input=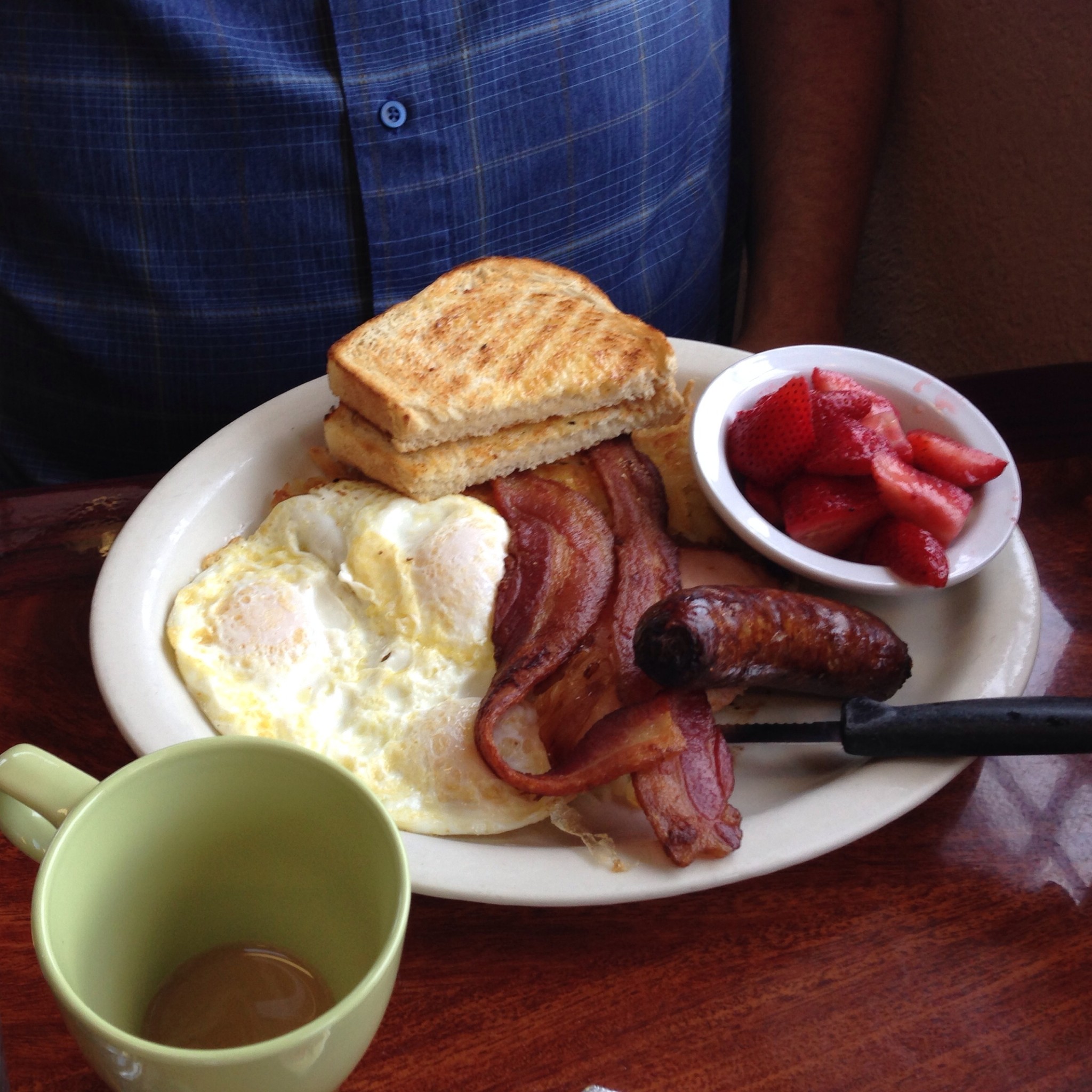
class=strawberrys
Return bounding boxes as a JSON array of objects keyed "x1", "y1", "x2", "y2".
[{"x1": 727, "y1": 365, "x2": 1009, "y2": 587}]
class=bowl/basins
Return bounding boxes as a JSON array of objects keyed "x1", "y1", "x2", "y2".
[{"x1": 688, "y1": 343, "x2": 1022, "y2": 596}]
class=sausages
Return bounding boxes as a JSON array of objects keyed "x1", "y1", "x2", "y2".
[{"x1": 632, "y1": 583, "x2": 913, "y2": 701}]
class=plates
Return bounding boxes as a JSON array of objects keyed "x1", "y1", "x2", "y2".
[{"x1": 88, "y1": 333, "x2": 1042, "y2": 908}]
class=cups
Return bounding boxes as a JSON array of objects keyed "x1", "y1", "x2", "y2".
[{"x1": 0, "y1": 735, "x2": 412, "y2": 1092}]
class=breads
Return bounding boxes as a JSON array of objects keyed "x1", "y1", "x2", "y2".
[{"x1": 322, "y1": 258, "x2": 687, "y2": 503}]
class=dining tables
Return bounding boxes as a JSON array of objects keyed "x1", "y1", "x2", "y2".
[{"x1": 0, "y1": 367, "x2": 1092, "y2": 1092}]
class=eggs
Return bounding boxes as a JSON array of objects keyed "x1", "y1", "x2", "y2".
[{"x1": 166, "y1": 481, "x2": 585, "y2": 836}]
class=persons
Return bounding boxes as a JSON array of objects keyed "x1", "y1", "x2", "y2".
[{"x1": 0, "y1": 0, "x2": 729, "y2": 491}]
